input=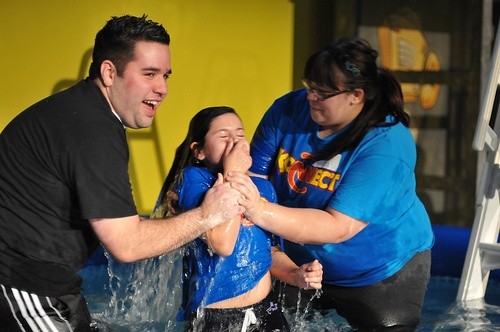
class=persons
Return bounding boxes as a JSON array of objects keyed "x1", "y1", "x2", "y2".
[
  {"x1": 0, "y1": 14, "x2": 247, "y2": 332},
  {"x1": 153, "y1": 106, "x2": 323, "y2": 332},
  {"x1": 224, "y1": 37, "x2": 435, "y2": 332}
]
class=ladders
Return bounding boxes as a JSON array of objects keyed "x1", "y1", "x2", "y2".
[{"x1": 455, "y1": 32, "x2": 500, "y2": 303}]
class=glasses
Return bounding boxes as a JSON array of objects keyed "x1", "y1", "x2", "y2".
[{"x1": 302, "y1": 79, "x2": 355, "y2": 100}]
